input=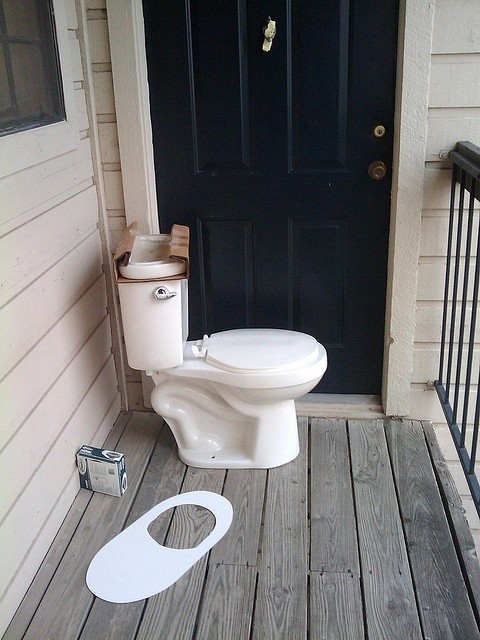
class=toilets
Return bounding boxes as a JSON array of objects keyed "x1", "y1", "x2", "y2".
[{"x1": 112, "y1": 222, "x2": 327, "y2": 469}]
